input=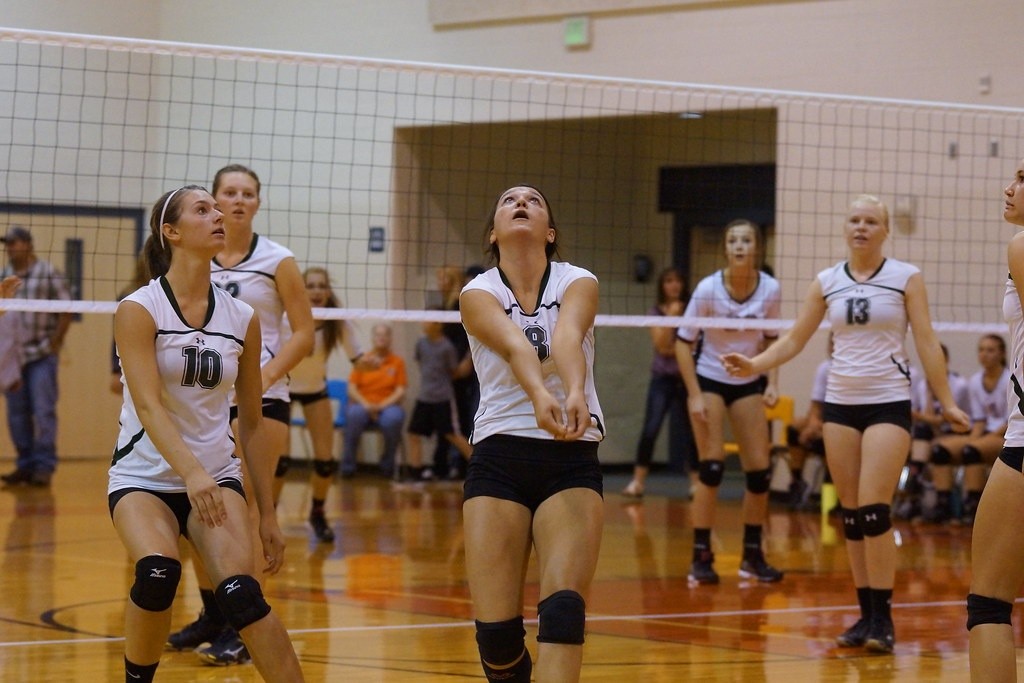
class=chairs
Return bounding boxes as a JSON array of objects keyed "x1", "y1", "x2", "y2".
[
  {"x1": 289, "y1": 383, "x2": 348, "y2": 465},
  {"x1": 724, "y1": 394, "x2": 967, "y2": 525}
]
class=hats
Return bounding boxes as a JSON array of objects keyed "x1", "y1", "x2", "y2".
[{"x1": 0, "y1": 227, "x2": 31, "y2": 242}]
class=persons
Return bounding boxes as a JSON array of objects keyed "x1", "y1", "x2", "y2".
[
  {"x1": 965, "y1": 156, "x2": 1024, "y2": 681},
  {"x1": 898, "y1": 333, "x2": 1011, "y2": 525},
  {"x1": 675, "y1": 219, "x2": 784, "y2": 584},
  {"x1": 107, "y1": 185, "x2": 307, "y2": 683},
  {"x1": 621, "y1": 264, "x2": 703, "y2": 496},
  {"x1": 787, "y1": 325, "x2": 833, "y2": 511},
  {"x1": 407, "y1": 305, "x2": 474, "y2": 478},
  {"x1": 431, "y1": 265, "x2": 487, "y2": 479},
  {"x1": 274, "y1": 259, "x2": 381, "y2": 542},
  {"x1": 718, "y1": 194, "x2": 973, "y2": 655},
  {"x1": 459, "y1": 185, "x2": 606, "y2": 683},
  {"x1": 111, "y1": 246, "x2": 151, "y2": 395},
  {"x1": 166, "y1": 164, "x2": 315, "y2": 659},
  {"x1": 339, "y1": 321, "x2": 407, "y2": 480},
  {"x1": 0, "y1": 226, "x2": 77, "y2": 488}
]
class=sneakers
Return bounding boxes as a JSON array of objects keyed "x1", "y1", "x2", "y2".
[
  {"x1": 688, "y1": 562, "x2": 719, "y2": 585},
  {"x1": 165, "y1": 608, "x2": 224, "y2": 652},
  {"x1": 738, "y1": 558, "x2": 784, "y2": 583},
  {"x1": 836, "y1": 617, "x2": 869, "y2": 648},
  {"x1": 196, "y1": 629, "x2": 253, "y2": 667},
  {"x1": 866, "y1": 616, "x2": 894, "y2": 650}
]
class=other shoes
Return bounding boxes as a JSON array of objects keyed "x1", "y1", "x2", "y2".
[
  {"x1": 911, "y1": 502, "x2": 952, "y2": 527},
  {"x1": 309, "y1": 511, "x2": 334, "y2": 544},
  {"x1": 895, "y1": 499, "x2": 921, "y2": 521},
  {"x1": 33, "y1": 463, "x2": 52, "y2": 486},
  {"x1": 1, "y1": 467, "x2": 32, "y2": 485},
  {"x1": 952, "y1": 505, "x2": 975, "y2": 528}
]
